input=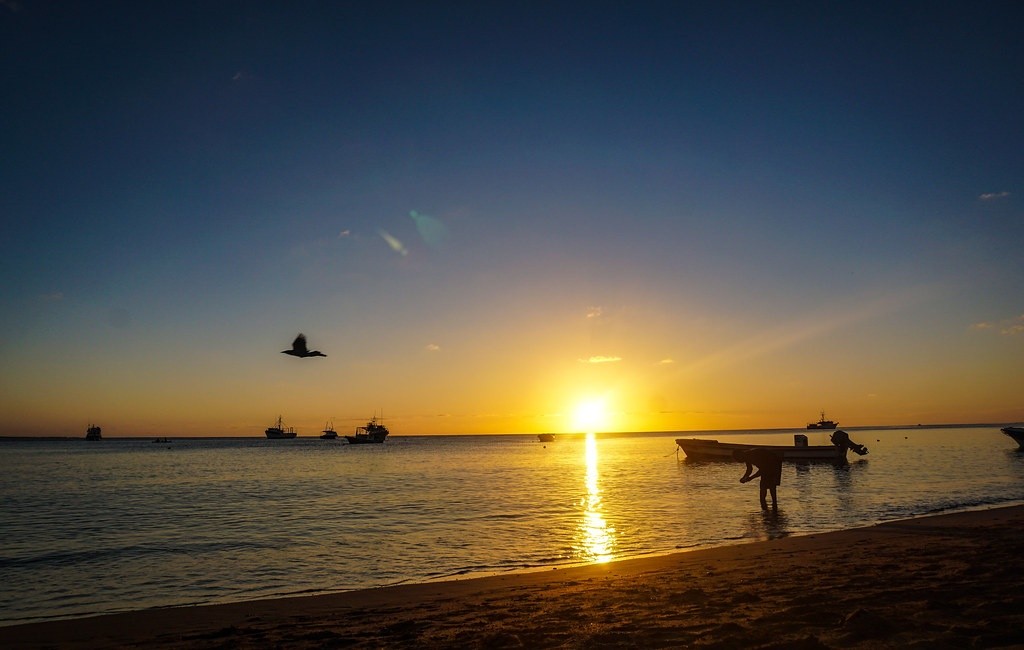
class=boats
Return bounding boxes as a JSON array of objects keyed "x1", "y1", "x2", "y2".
[
  {"x1": 264, "y1": 415, "x2": 297, "y2": 439},
  {"x1": 538, "y1": 433, "x2": 555, "y2": 442},
  {"x1": 152, "y1": 441, "x2": 172, "y2": 443},
  {"x1": 1001, "y1": 427, "x2": 1024, "y2": 451},
  {"x1": 675, "y1": 439, "x2": 848, "y2": 466}
]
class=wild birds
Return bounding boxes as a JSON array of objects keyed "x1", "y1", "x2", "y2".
[{"x1": 280, "y1": 332, "x2": 328, "y2": 358}]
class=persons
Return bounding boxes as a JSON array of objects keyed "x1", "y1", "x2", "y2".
[{"x1": 733, "y1": 447, "x2": 782, "y2": 510}]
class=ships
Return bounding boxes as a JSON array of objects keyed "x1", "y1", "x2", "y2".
[
  {"x1": 86, "y1": 423, "x2": 102, "y2": 441},
  {"x1": 344, "y1": 408, "x2": 389, "y2": 444},
  {"x1": 807, "y1": 411, "x2": 839, "y2": 430},
  {"x1": 319, "y1": 420, "x2": 338, "y2": 439}
]
